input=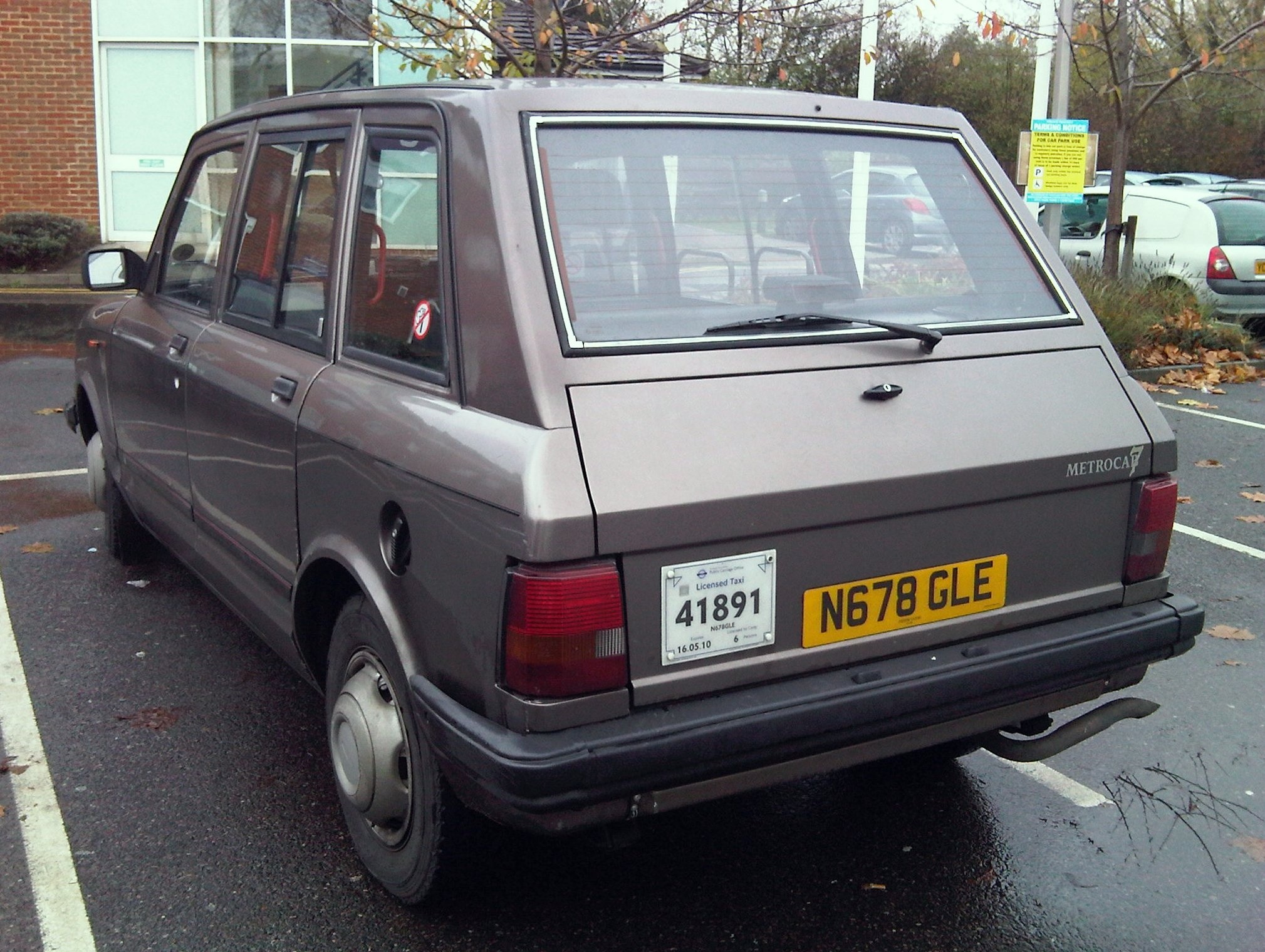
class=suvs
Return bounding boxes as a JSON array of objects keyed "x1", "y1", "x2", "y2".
[
  {"x1": 65, "y1": 78, "x2": 1204, "y2": 911},
  {"x1": 1036, "y1": 186, "x2": 1265, "y2": 345}
]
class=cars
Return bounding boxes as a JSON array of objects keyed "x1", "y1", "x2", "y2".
[
  {"x1": 773, "y1": 163, "x2": 952, "y2": 255},
  {"x1": 1083, "y1": 169, "x2": 1265, "y2": 204}
]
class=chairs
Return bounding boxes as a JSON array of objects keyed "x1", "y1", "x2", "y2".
[{"x1": 548, "y1": 167, "x2": 635, "y2": 298}]
